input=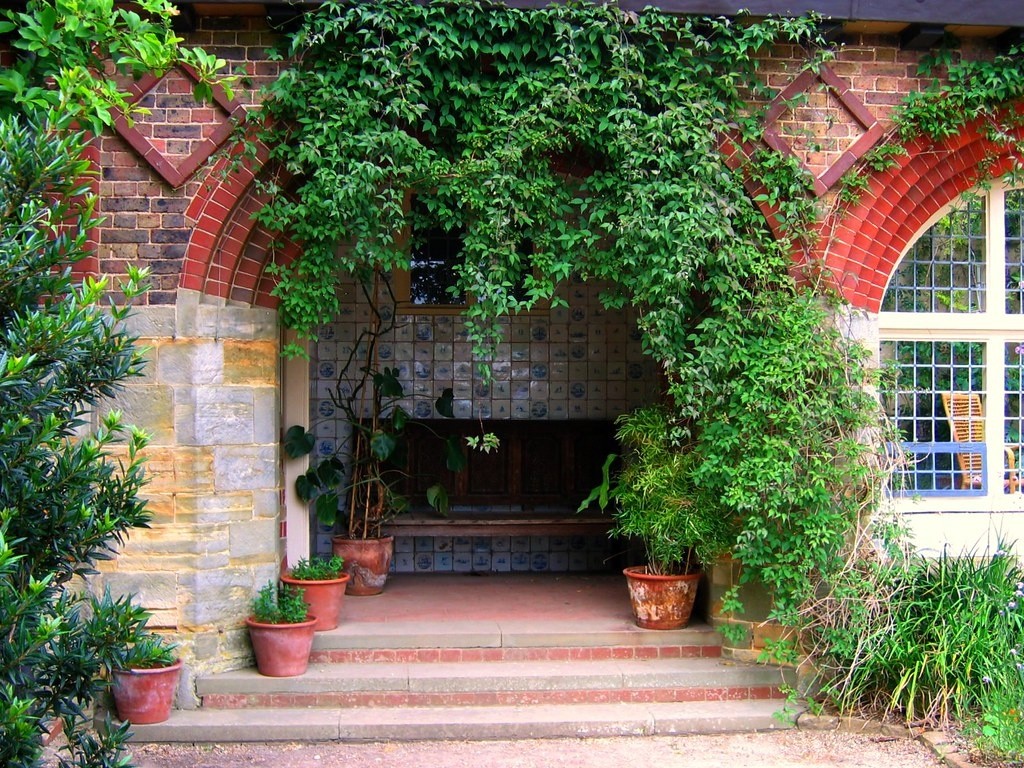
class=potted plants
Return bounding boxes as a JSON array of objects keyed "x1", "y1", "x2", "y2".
[
  {"x1": 284, "y1": 272, "x2": 466, "y2": 596},
  {"x1": 605, "y1": 401, "x2": 741, "y2": 630},
  {"x1": 281, "y1": 553, "x2": 350, "y2": 630},
  {"x1": 244, "y1": 579, "x2": 318, "y2": 677}
]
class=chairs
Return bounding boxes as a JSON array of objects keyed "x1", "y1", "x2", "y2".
[{"x1": 943, "y1": 393, "x2": 1024, "y2": 495}]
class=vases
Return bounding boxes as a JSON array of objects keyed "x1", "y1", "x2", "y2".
[{"x1": 112, "y1": 659, "x2": 182, "y2": 723}]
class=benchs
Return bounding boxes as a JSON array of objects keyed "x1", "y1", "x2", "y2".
[{"x1": 351, "y1": 416, "x2": 616, "y2": 538}]
